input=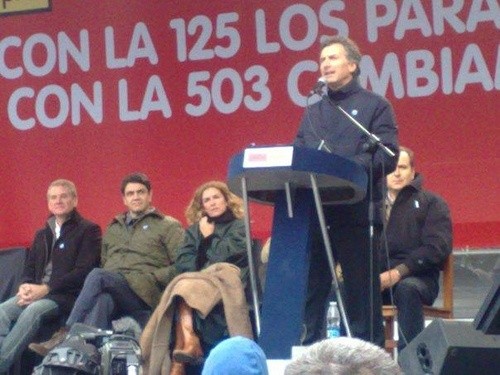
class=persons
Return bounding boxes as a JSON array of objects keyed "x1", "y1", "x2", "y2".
[
  {"x1": 168, "y1": 181, "x2": 254, "y2": 375},
  {"x1": 294, "y1": 36, "x2": 399, "y2": 346},
  {"x1": 201, "y1": 336, "x2": 405, "y2": 375},
  {"x1": 337, "y1": 146, "x2": 454, "y2": 354},
  {"x1": 0, "y1": 179, "x2": 102, "y2": 375},
  {"x1": 28, "y1": 172, "x2": 186, "y2": 356}
]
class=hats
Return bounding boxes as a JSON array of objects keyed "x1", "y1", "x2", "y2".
[{"x1": 201, "y1": 336, "x2": 268, "y2": 375}]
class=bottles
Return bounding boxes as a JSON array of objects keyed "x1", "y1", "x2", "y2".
[{"x1": 327, "y1": 300, "x2": 340, "y2": 338}]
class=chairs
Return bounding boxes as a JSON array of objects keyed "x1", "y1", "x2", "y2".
[
  {"x1": 0, "y1": 237, "x2": 261, "y2": 375},
  {"x1": 380, "y1": 252, "x2": 457, "y2": 354}
]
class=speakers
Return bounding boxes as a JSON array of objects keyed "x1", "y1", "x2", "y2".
[{"x1": 397, "y1": 319, "x2": 500, "y2": 375}]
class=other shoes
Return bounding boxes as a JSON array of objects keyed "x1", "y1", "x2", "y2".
[{"x1": 29, "y1": 327, "x2": 67, "y2": 357}]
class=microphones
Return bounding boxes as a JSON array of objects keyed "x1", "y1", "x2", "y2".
[{"x1": 309, "y1": 77, "x2": 327, "y2": 97}]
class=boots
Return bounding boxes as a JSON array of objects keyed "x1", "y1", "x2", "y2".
[
  {"x1": 168, "y1": 312, "x2": 185, "y2": 375},
  {"x1": 172, "y1": 300, "x2": 204, "y2": 366}
]
